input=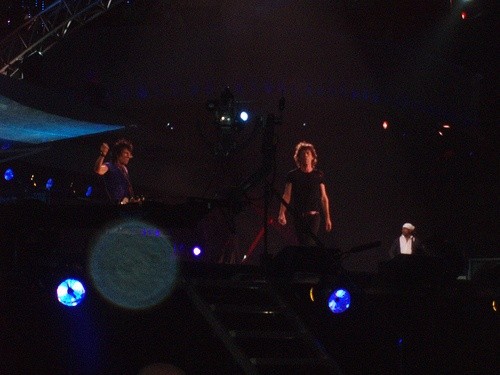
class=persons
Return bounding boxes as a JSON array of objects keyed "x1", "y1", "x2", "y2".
[
  {"x1": 388, "y1": 221, "x2": 426, "y2": 259},
  {"x1": 93, "y1": 139, "x2": 139, "y2": 209},
  {"x1": 278, "y1": 141, "x2": 333, "y2": 247}
]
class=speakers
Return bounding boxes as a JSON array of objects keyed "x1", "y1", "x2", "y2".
[{"x1": 274, "y1": 246, "x2": 335, "y2": 275}]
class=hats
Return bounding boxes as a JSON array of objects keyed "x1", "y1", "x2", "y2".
[{"x1": 402, "y1": 223, "x2": 415, "y2": 231}]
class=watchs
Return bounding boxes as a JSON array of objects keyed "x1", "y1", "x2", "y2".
[{"x1": 99, "y1": 151, "x2": 107, "y2": 156}]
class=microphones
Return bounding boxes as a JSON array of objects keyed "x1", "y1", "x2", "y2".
[{"x1": 278, "y1": 96, "x2": 287, "y2": 121}]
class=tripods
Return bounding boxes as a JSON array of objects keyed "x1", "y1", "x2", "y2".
[{"x1": 219, "y1": 115, "x2": 329, "y2": 269}]
